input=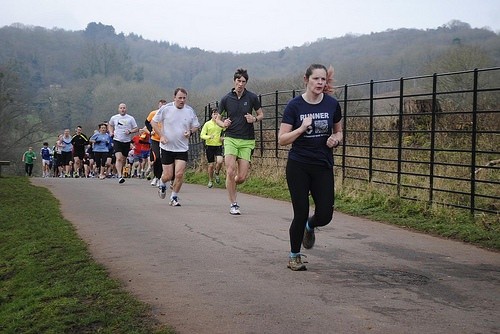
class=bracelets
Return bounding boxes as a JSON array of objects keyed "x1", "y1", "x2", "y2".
[
  {"x1": 334, "y1": 141, "x2": 339, "y2": 148},
  {"x1": 254, "y1": 116, "x2": 257, "y2": 121},
  {"x1": 190, "y1": 130, "x2": 193, "y2": 136},
  {"x1": 129, "y1": 130, "x2": 131, "y2": 134}
]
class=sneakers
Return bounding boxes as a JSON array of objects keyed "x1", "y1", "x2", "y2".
[
  {"x1": 158, "y1": 178, "x2": 166, "y2": 199},
  {"x1": 230, "y1": 202, "x2": 241, "y2": 215},
  {"x1": 287, "y1": 253, "x2": 309, "y2": 271},
  {"x1": 303, "y1": 217, "x2": 315, "y2": 249},
  {"x1": 169, "y1": 195, "x2": 181, "y2": 206}
]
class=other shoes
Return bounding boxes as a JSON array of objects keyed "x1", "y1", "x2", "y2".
[
  {"x1": 53, "y1": 172, "x2": 118, "y2": 180},
  {"x1": 151, "y1": 177, "x2": 158, "y2": 185},
  {"x1": 207, "y1": 180, "x2": 213, "y2": 188},
  {"x1": 169, "y1": 180, "x2": 173, "y2": 189},
  {"x1": 119, "y1": 178, "x2": 125, "y2": 183},
  {"x1": 156, "y1": 179, "x2": 160, "y2": 188},
  {"x1": 214, "y1": 170, "x2": 221, "y2": 185}
]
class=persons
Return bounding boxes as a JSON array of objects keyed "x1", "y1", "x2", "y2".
[
  {"x1": 150, "y1": 87, "x2": 199, "y2": 207},
  {"x1": 40, "y1": 120, "x2": 154, "y2": 180},
  {"x1": 108, "y1": 102, "x2": 138, "y2": 183},
  {"x1": 216, "y1": 68, "x2": 263, "y2": 214},
  {"x1": 278, "y1": 64, "x2": 343, "y2": 270},
  {"x1": 200, "y1": 108, "x2": 226, "y2": 187},
  {"x1": 145, "y1": 100, "x2": 175, "y2": 189},
  {"x1": 22, "y1": 147, "x2": 36, "y2": 176},
  {"x1": 89, "y1": 123, "x2": 110, "y2": 178}
]
